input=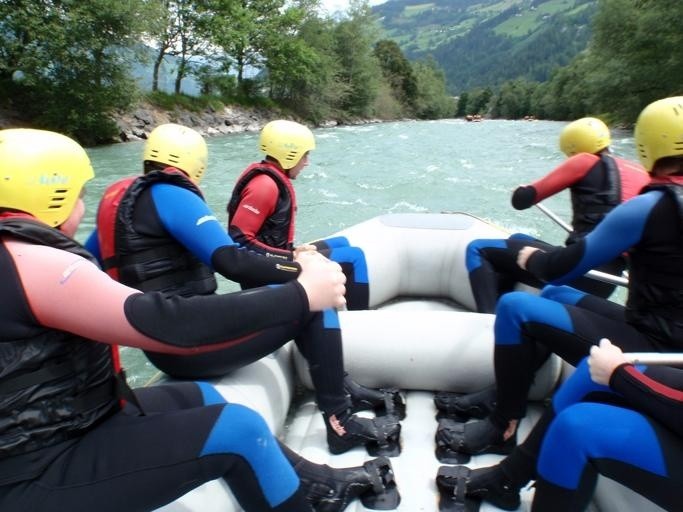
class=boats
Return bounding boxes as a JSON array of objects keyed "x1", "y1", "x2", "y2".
[
  {"x1": 472, "y1": 118, "x2": 480, "y2": 122},
  {"x1": 132, "y1": 206, "x2": 671, "y2": 511},
  {"x1": 528, "y1": 119, "x2": 532, "y2": 121}
]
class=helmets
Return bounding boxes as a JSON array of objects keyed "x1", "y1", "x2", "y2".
[
  {"x1": 260, "y1": 119, "x2": 315, "y2": 170},
  {"x1": 634, "y1": 96, "x2": 683, "y2": 172},
  {"x1": 142, "y1": 123, "x2": 208, "y2": 182},
  {"x1": 0, "y1": 128, "x2": 96, "y2": 227},
  {"x1": 559, "y1": 117, "x2": 610, "y2": 158}
]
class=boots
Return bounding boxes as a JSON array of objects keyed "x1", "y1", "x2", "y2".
[
  {"x1": 322, "y1": 402, "x2": 399, "y2": 455},
  {"x1": 344, "y1": 375, "x2": 401, "y2": 411},
  {"x1": 439, "y1": 409, "x2": 521, "y2": 455},
  {"x1": 439, "y1": 448, "x2": 538, "y2": 511},
  {"x1": 435, "y1": 384, "x2": 496, "y2": 418},
  {"x1": 293, "y1": 458, "x2": 389, "y2": 512}
]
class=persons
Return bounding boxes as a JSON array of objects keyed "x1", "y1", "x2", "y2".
[
  {"x1": 226, "y1": 119, "x2": 371, "y2": 310},
  {"x1": 438, "y1": 342, "x2": 682, "y2": 512},
  {"x1": 1, "y1": 126, "x2": 383, "y2": 512},
  {"x1": 81, "y1": 125, "x2": 401, "y2": 456},
  {"x1": 436, "y1": 97, "x2": 683, "y2": 454},
  {"x1": 467, "y1": 116, "x2": 651, "y2": 313}
]
